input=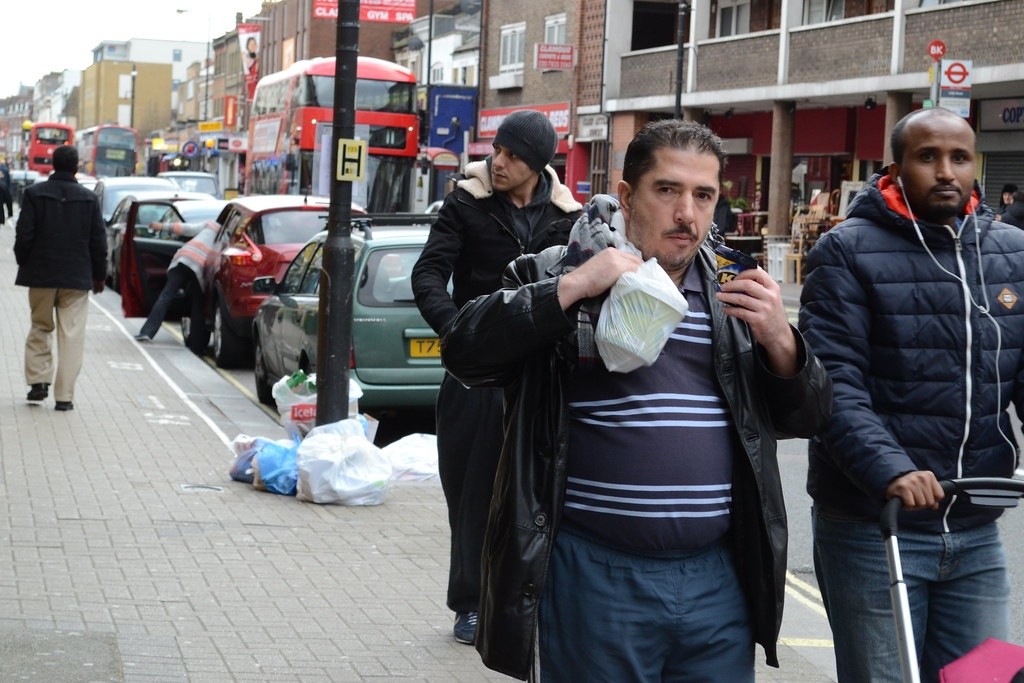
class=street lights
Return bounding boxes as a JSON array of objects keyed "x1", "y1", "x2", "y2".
[{"x1": 176, "y1": 8, "x2": 212, "y2": 173}]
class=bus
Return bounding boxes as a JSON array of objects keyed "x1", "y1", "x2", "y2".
[
  {"x1": 27, "y1": 122, "x2": 73, "y2": 174},
  {"x1": 77, "y1": 124, "x2": 139, "y2": 178},
  {"x1": 244, "y1": 56, "x2": 429, "y2": 225}
]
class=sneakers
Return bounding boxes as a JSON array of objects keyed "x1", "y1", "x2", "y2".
[{"x1": 454, "y1": 612, "x2": 478, "y2": 644}]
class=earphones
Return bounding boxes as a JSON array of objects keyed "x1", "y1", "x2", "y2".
[{"x1": 897, "y1": 176, "x2": 904, "y2": 187}]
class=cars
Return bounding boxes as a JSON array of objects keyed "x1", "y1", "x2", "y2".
[
  {"x1": 6, "y1": 169, "x2": 98, "y2": 201},
  {"x1": 156, "y1": 170, "x2": 225, "y2": 201},
  {"x1": 102, "y1": 192, "x2": 215, "y2": 296},
  {"x1": 91, "y1": 177, "x2": 181, "y2": 224},
  {"x1": 118, "y1": 193, "x2": 366, "y2": 370},
  {"x1": 134, "y1": 197, "x2": 228, "y2": 244},
  {"x1": 254, "y1": 212, "x2": 455, "y2": 420}
]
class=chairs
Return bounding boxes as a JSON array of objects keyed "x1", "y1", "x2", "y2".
[{"x1": 785, "y1": 188, "x2": 846, "y2": 284}]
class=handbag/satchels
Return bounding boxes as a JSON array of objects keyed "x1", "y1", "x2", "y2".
[
  {"x1": 229, "y1": 371, "x2": 392, "y2": 506},
  {"x1": 594, "y1": 243, "x2": 688, "y2": 373}
]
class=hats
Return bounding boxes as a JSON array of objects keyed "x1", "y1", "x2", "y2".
[{"x1": 492, "y1": 110, "x2": 558, "y2": 172}]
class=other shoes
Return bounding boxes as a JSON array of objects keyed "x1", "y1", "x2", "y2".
[
  {"x1": 27, "y1": 383, "x2": 48, "y2": 401},
  {"x1": 135, "y1": 334, "x2": 151, "y2": 342},
  {"x1": 55, "y1": 401, "x2": 74, "y2": 412}
]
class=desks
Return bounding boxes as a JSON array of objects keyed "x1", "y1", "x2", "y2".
[{"x1": 723, "y1": 235, "x2": 762, "y2": 251}]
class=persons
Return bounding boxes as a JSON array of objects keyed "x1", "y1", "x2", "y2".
[
  {"x1": 964, "y1": 179, "x2": 982, "y2": 214},
  {"x1": 435, "y1": 119, "x2": 834, "y2": 683},
  {"x1": 410, "y1": 110, "x2": 583, "y2": 643},
  {"x1": 995, "y1": 184, "x2": 1024, "y2": 230},
  {"x1": 135, "y1": 220, "x2": 232, "y2": 353},
  {"x1": 13, "y1": 144, "x2": 109, "y2": 410},
  {"x1": 0, "y1": 170, "x2": 13, "y2": 224},
  {"x1": 797, "y1": 107, "x2": 1024, "y2": 683},
  {"x1": 245, "y1": 37, "x2": 257, "y2": 67}
]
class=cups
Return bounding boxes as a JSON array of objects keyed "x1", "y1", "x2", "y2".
[{"x1": 597, "y1": 278, "x2": 689, "y2": 367}]
